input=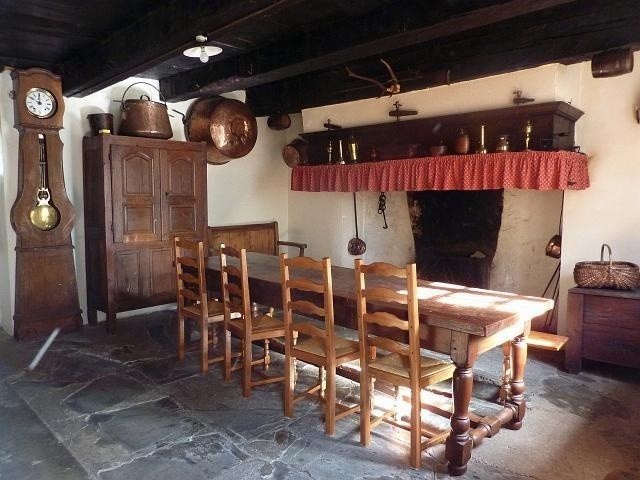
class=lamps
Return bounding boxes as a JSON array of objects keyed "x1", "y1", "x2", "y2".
[{"x1": 182, "y1": 35, "x2": 251, "y2": 63}]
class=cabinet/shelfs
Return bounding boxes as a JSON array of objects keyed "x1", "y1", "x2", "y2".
[
  {"x1": 562, "y1": 280, "x2": 640, "y2": 375},
  {"x1": 82, "y1": 133, "x2": 208, "y2": 336}
]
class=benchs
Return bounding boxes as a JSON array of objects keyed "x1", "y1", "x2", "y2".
[{"x1": 499, "y1": 329, "x2": 568, "y2": 404}]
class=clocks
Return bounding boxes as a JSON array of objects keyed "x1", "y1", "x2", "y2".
[{"x1": 10, "y1": 67, "x2": 66, "y2": 130}]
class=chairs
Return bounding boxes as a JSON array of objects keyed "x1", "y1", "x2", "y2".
[
  {"x1": 279, "y1": 252, "x2": 377, "y2": 437},
  {"x1": 218, "y1": 241, "x2": 299, "y2": 397},
  {"x1": 354, "y1": 258, "x2": 458, "y2": 467},
  {"x1": 207, "y1": 220, "x2": 307, "y2": 258},
  {"x1": 173, "y1": 235, "x2": 251, "y2": 373}
]
